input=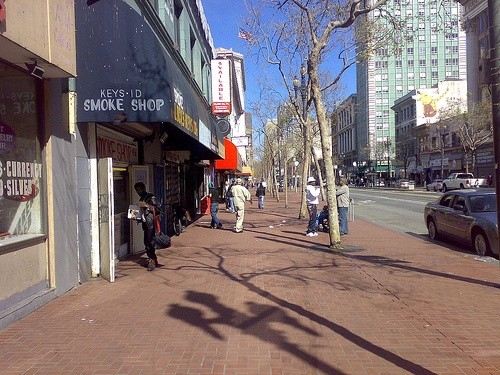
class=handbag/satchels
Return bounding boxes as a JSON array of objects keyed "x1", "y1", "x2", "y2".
[{"x1": 154, "y1": 231, "x2": 171, "y2": 249}]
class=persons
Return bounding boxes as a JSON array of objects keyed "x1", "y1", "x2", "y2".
[
  {"x1": 223, "y1": 181, "x2": 232, "y2": 211},
  {"x1": 336, "y1": 178, "x2": 349, "y2": 235},
  {"x1": 135, "y1": 183, "x2": 161, "y2": 271},
  {"x1": 262, "y1": 180, "x2": 267, "y2": 195},
  {"x1": 232, "y1": 178, "x2": 251, "y2": 232},
  {"x1": 391, "y1": 174, "x2": 401, "y2": 182},
  {"x1": 207, "y1": 183, "x2": 223, "y2": 229},
  {"x1": 383, "y1": 174, "x2": 387, "y2": 184},
  {"x1": 228, "y1": 180, "x2": 236, "y2": 213},
  {"x1": 256, "y1": 183, "x2": 265, "y2": 209},
  {"x1": 425, "y1": 173, "x2": 432, "y2": 191},
  {"x1": 306, "y1": 176, "x2": 322, "y2": 237},
  {"x1": 488, "y1": 173, "x2": 493, "y2": 187}
]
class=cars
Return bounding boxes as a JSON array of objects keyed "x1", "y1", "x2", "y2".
[
  {"x1": 370, "y1": 179, "x2": 415, "y2": 188},
  {"x1": 423, "y1": 190, "x2": 500, "y2": 258},
  {"x1": 426, "y1": 178, "x2": 444, "y2": 192}
]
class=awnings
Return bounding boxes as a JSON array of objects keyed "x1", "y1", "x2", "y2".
[
  {"x1": 214, "y1": 138, "x2": 242, "y2": 173},
  {"x1": 74, "y1": 0, "x2": 224, "y2": 160},
  {"x1": 236, "y1": 165, "x2": 252, "y2": 176}
]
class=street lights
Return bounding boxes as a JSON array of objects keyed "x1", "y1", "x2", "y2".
[
  {"x1": 384, "y1": 135, "x2": 392, "y2": 179},
  {"x1": 293, "y1": 64, "x2": 308, "y2": 138}
]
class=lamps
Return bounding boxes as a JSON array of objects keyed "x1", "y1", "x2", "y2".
[
  {"x1": 158, "y1": 131, "x2": 168, "y2": 143},
  {"x1": 27, "y1": 59, "x2": 45, "y2": 80}
]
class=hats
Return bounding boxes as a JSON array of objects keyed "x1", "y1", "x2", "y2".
[
  {"x1": 208, "y1": 182, "x2": 214, "y2": 188},
  {"x1": 307, "y1": 177, "x2": 316, "y2": 182},
  {"x1": 236, "y1": 178, "x2": 242, "y2": 184}
]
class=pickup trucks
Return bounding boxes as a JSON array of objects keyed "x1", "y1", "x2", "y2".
[{"x1": 442, "y1": 172, "x2": 489, "y2": 192}]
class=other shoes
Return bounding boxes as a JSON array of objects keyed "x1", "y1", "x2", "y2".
[
  {"x1": 340, "y1": 233, "x2": 345, "y2": 236},
  {"x1": 306, "y1": 232, "x2": 315, "y2": 236},
  {"x1": 211, "y1": 223, "x2": 215, "y2": 229},
  {"x1": 147, "y1": 258, "x2": 155, "y2": 270},
  {"x1": 236, "y1": 230, "x2": 243, "y2": 232},
  {"x1": 313, "y1": 232, "x2": 318, "y2": 236},
  {"x1": 216, "y1": 222, "x2": 222, "y2": 229}
]
